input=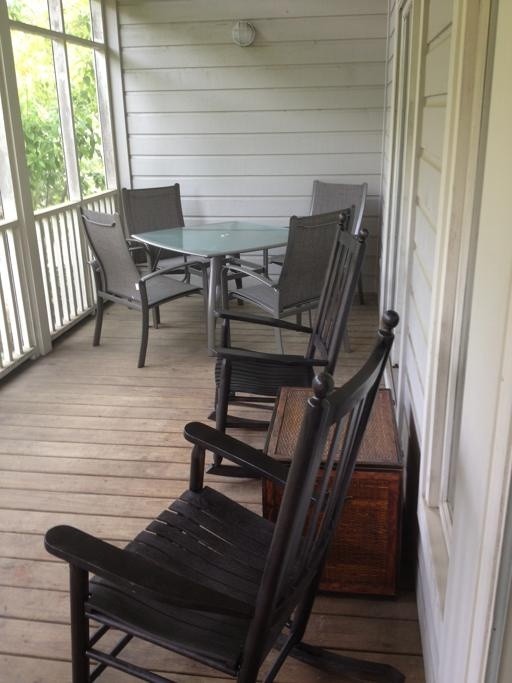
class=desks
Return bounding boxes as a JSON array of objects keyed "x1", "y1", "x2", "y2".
[{"x1": 262, "y1": 385, "x2": 403, "y2": 598}]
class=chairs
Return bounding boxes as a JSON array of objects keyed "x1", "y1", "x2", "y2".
[
  {"x1": 209, "y1": 212, "x2": 368, "y2": 479},
  {"x1": 44, "y1": 311, "x2": 406, "y2": 683}
]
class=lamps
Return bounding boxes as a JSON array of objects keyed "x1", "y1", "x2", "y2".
[{"x1": 232, "y1": 21, "x2": 256, "y2": 47}]
